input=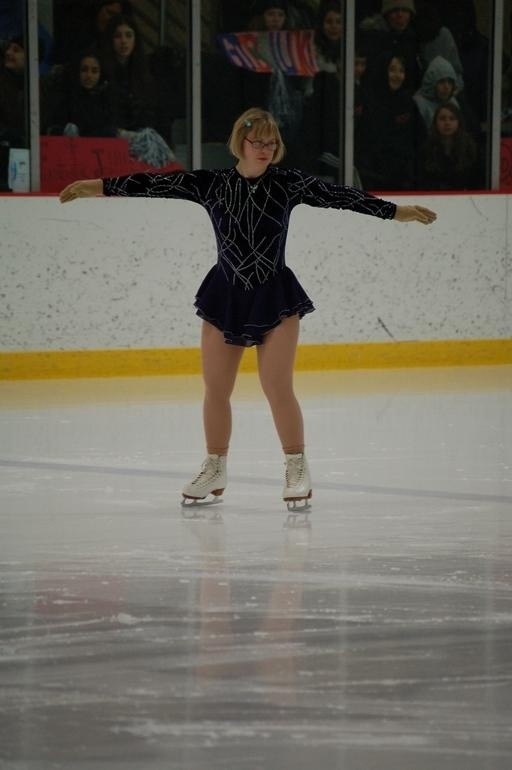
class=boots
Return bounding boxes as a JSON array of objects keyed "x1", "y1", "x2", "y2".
[
  {"x1": 283, "y1": 452, "x2": 312, "y2": 500},
  {"x1": 183, "y1": 454, "x2": 228, "y2": 499}
]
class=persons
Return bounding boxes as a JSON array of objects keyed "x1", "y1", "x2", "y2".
[
  {"x1": 231, "y1": 1, "x2": 490, "y2": 193},
  {"x1": 58, "y1": 108, "x2": 438, "y2": 512},
  {"x1": 1, "y1": 0, "x2": 187, "y2": 168}
]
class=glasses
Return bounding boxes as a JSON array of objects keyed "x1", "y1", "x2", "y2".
[{"x1": 243, "y1": 137, "x2": 279, "y2": 152}]
